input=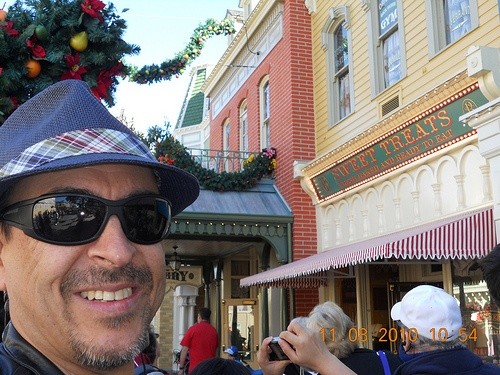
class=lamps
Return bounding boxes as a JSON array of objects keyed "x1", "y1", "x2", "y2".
[{"x1": 167, "y1": 251, "x2": 182, "y2": 272}]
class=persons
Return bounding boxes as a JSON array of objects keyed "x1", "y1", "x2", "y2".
[
  {"x1": 179, "y1": 307, "x2": 219, "y2": 374},
  {"x1": 132, "y1": 321, "x2": 170, "y2": 375},
  {"x1": 256, "y1": 321, "x2": 357, "y2": 374},
  {"x1": 481, "y1": 243, "x2": 500, "y2": 311},
  {"x1": 225, "y1": 346, "x2": 242, "y2": 364},
  {"x1": 0, "y1": 79, "x2": 200, "y2": 375},
  {"x1": 391, "y1": 285, "x2": 500, "y2": 375},
  {"x1": 297, "y1": 301, "x2": 403, "y2": 375}
]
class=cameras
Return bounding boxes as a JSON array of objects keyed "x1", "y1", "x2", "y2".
[{"x1": 269, "y1": 337, "x2": 296, "y2": 360}]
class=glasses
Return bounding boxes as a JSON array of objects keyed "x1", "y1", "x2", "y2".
[
  {"x1": 154, "y1": 333, "x2": 160, "y2": 339},
  {"x1": 0, "y1": 192, "x2": 173, "y2": 246}
]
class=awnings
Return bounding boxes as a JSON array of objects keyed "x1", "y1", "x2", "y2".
[{"x1": 239, "y1": 206, "x2": 496, "y2": 288}]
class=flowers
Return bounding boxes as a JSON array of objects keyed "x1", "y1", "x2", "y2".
[
  {"x1": 6, "y1": 97, "x2": 19, "y2": 111},
  {"x1": 91, "y1": 61, "x2": 125, "y2": 101},
  {"x1": 61, "y1": 55, "x2": 86, "y2": 81},
  {"x1": 26, "y1": 40, "x2": 45, "y2": 58},
  {"x1": 0, "y1": 21, "x2": 19, "y2": 37},
  {"x1": 81, "y1": 0, "x2": 106, "y2": 22},
  {"x1": 241, "y1": 148, "x2": 277, "y2": 171},
  {"x1": 0, "y1": 68, "x2": 3, "y2": 75},
  {"x1": 156, "y1": 155, "x2": 175, "y2": 165}
]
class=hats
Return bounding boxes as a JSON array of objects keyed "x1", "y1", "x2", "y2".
[
  {"x1": 1, "y1": 78, "x2": 200, "y2": 217},
  {"x1": 391, "y1": 284, "x2": 462, "y2": 342},
  {"x1": 224, "y1": 346, "x2": 239, "y2": 355}
]
